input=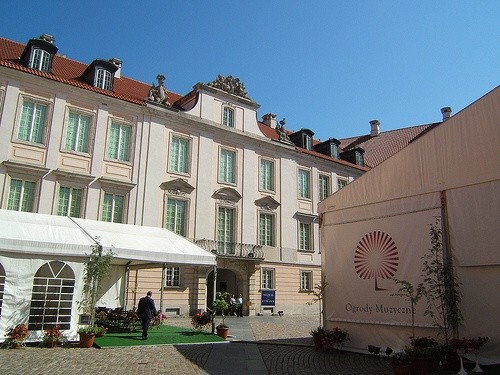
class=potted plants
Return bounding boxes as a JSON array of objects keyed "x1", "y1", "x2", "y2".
[
  {"x1": 440, "y1": 262, "x2": 465, "y2": 368},
  {"x1": 392, "y1": 337, "x2": 439, "y2": 375},
  {"x1": 78, "y1": 327, "x2": 103, "y2": 349},
  {"x1": 213, "y1": 291, "x2": 240, "y2": 339}
]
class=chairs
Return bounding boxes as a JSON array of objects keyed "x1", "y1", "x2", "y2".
[{"x1": 96, "y1": 308, "x2": 162, "y2": 332}]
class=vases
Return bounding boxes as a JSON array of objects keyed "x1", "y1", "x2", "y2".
[
  {"x1": 414, "y1": 344, "x2": 425, "y2": 352},
  {"x1": 45, "y1": 340, "x2": 57, "y2": 349},
  {"x1": 456, "y1": 349, "x2": 469, "y2": 375},
  {"x1": 13, "y1": 343, "x2": 22, "y2": 349},
  {"x1": 313, "y1": 337, "x2": 333, "y2": 350},
  {"x1": 472, "y1": 349, "x2": 485, "y2": 373}
]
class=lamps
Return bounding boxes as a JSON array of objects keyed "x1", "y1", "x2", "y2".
[
  {"x1": 384, "y1": 348, "x2": 393, "y2": 360},
  {"x1": 374, "y1": 348, "x2": 382, "y2": 360},
  {"x1": 369, "y1": 345, "x2": 375, "y2": 358}
]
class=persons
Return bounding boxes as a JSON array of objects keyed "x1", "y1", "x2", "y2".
[
  {"x1": 229, "y1": 293, "x2": 236, "y2": 316},
  {"x1": 137, "y1": 291, "x2": 159, "y2": 340},
  {"x1": 236, "y1": 293, "x2": 243, "y2": 317}
]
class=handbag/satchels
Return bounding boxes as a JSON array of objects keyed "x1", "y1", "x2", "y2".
[{"x1": 138, "y1": 309, "x2": 146, "y2": 318}]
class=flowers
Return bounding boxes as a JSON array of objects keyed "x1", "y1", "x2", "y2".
[
  {"x1": 42, "y1": 325, "x2": 65, "y2": 342},
  {"x1": 4, "y1": 324, "x2": 30, "y2": 342},
  {"x1": 313, "y1": 327, "x2": 346, "y2": 344},
  {"x1": 449, "y1": 336, "x2": 490, "y2": 349},
  {"x1": 409, "y1": 336, "x2": 438, "y2": 347}
]
